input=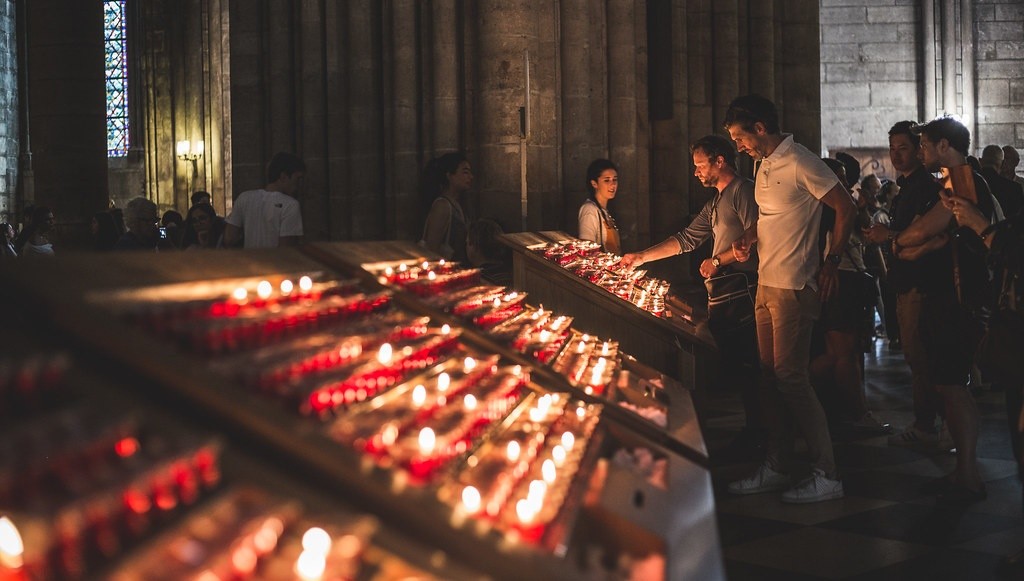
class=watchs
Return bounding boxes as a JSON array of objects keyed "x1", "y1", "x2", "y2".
[
  {"x1": 826, "y1": 254, "x2": 841, "y2": 263},
  {"x1": 712, "y1": 255, "x2": 723, "y2": 270}
]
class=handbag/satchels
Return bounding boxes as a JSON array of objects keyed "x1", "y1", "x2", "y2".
[
  {"x1": 954, "y1": 307, "x2": 985, "y2": 362},
  {"x1": 860, "y1": 272, "x2": 880, "y2": 307},
  {"x1": 973, "y1": 239, "x2": 1023, "y2": 390},
  {"x1": 703, "y1": 272, "x2": 755, "y2": 332},
  {"x1": 417, "y1": 198, "x2": 455, "y2": 259}
]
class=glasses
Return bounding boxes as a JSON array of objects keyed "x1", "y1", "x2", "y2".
[
  {"x1": 191, "y1": 215, "x2": 209, "y2": 224},
  {"x1": 138, "y1": 216, "x2": 161, "y2": 225}
]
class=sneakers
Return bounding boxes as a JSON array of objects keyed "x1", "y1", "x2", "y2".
[
  {"x1": 781, "y1": 469, "x2": 843, "y2": 503},
  {"x1": 887, "y1": 426, "x2": 938, "y2": 446},
  {"x1": 852, "y1": 411, "x2": 894, "y2": 433}
]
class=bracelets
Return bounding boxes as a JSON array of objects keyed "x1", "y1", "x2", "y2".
[
  {"x1": 888, "y1": 229, "x2": 894, "y2": 240},
  {"x1": 980, "y1": 226, "x2": 994, "y2": 241}
]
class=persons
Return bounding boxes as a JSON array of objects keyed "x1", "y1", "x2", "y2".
[
  {"x1": 618, "y1": 135, "x2": 767, "y2": 465},
  {"x1": 0, "y1": 152, "x2": 306, "y2": 259},
  {"x1": 422, "y1": 152, "x2": 513, "y2": 291},
  {"x1": 724, "y1": 95, "x2": 859, "y2": 504},
  {"x1": 809, "y1": 118, "x2": 1024, "y2": 493},
  {"x1": 578, "y1": 158, "x2": 621, "y2": 257}
]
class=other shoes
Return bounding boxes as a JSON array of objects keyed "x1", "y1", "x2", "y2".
[
  {"x1": 727, "y1": 459, "x2": 790, "y2": 496},
  {"x1": 918, "y1": 476, "x2": 952, "y2": 496},
  {"x1": 947, "y1": 479, "x2": 985, "y2": 514}
]
